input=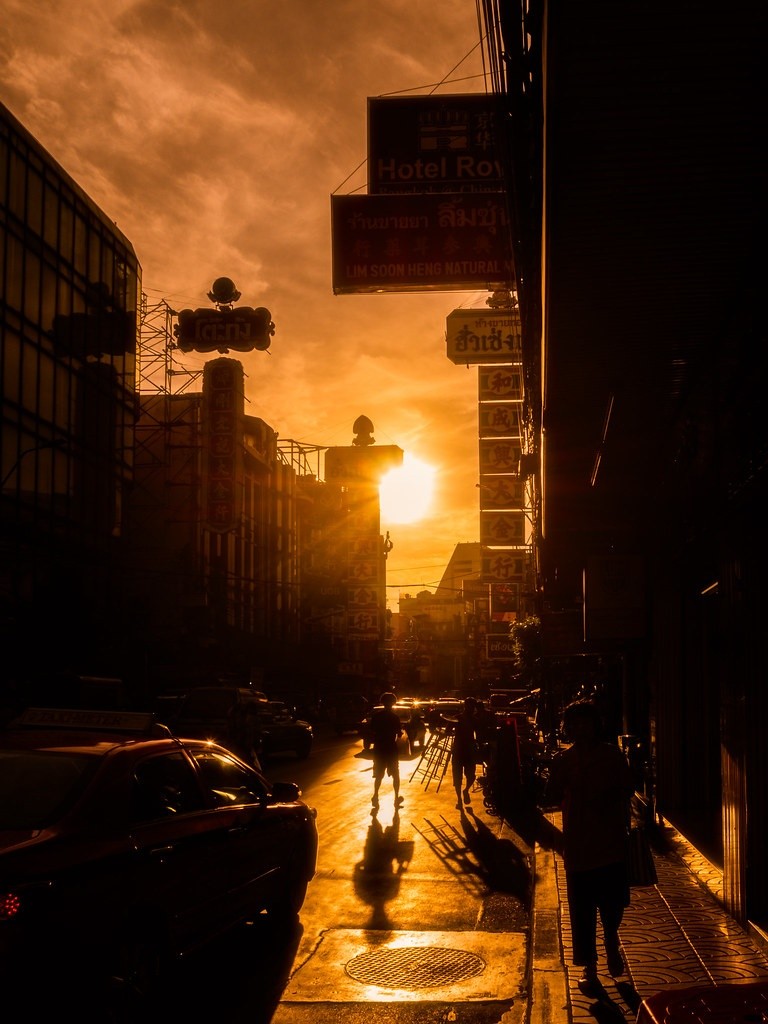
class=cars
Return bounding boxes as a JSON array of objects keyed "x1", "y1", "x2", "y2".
[
  {"x1": 1, "y1": 704, "x2": 316, "y2": 1008},
  {"x1": 354, "y1": 706, "x2": 427, "y2": 753},
  {"x1": 395, "y1": 697, "x2": 493, "y2": 731}
]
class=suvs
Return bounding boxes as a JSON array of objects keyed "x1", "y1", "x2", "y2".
[{"x1": 166, "y1": 686, "x2": 314, "y2": 766}]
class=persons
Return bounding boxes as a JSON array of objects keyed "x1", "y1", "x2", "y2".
[
  {"x1": 542, "y1": 700, "x2": 637, "y2": 995},
  {"x1": 364, "y1": 693, "x2": 403, "y2": 809},
  {"x1": 444, "y1": 698, "x2": 492, "y2": 809}
]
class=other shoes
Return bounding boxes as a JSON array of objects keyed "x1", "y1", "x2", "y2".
[
  {"x1": 578, "y1": 976, "x2": 603, "y2": 997},
  {"x1": 607, "y1": 948, "x2": 624, "y2": 977},
  {"x1": 463, "y1": 790, "x2": 470, "y2": 804}
]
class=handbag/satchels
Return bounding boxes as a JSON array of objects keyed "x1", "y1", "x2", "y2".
[
  {"x1": 395, "y1": 730, "x2": 412, "y2": 757},
  {"x1": 624, "y1": 827, "x2": 659, "y2": 886},
  {"x1": 453, "y1": 735, "x2": 490, "y2": 764}
]
